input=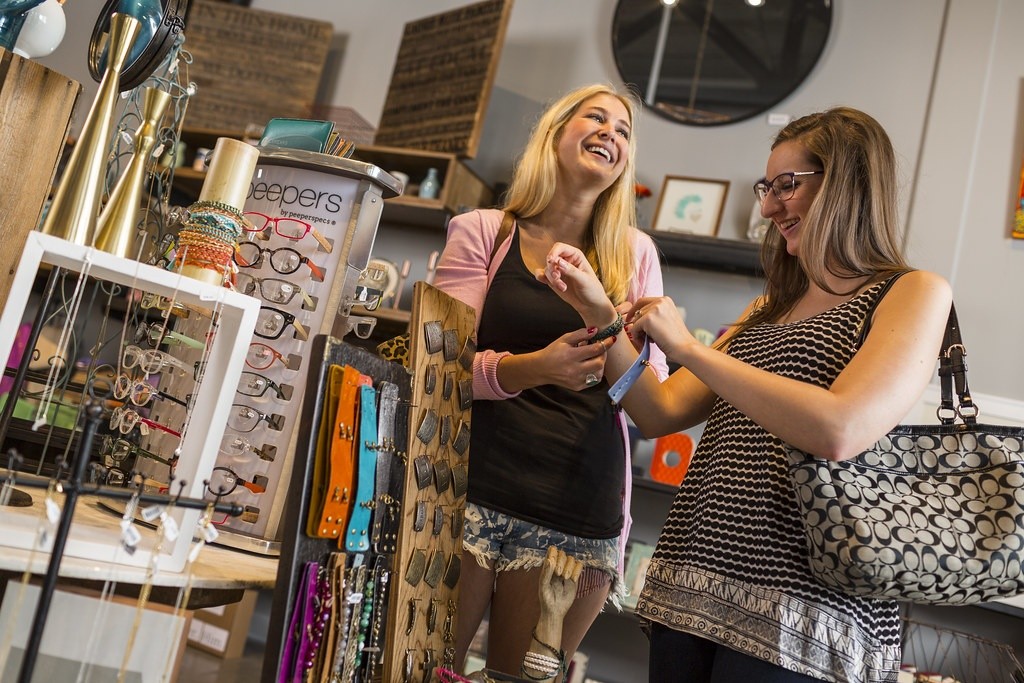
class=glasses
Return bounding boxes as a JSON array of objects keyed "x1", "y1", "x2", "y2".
[
  {"x1": 234, "y1": 272, "x2": 314, "y2": 307},
  {"x1": 186, "y1": 394, "x2": 279, "y2": 432},
  {"x1": 194, "y1": 360, "x2": 286, "y2": 400},
  {"x1": 752, "y1": 171, "x2": 824, "y2": 207},
  {"x1": 242, "y1": 212, "x2": 332, "y2": 254},
  {"x1": 232, "y1": 241, "x2": 324, "y2": 282},
  {"x1": 100, "y1": 434, "x2": 170, "y2": 466},
  {"x1": 122, "y1": 345, "x2": 195, "y2": 374},
  {"x1": 167, "y1": 458, "x2": 266, "y2": 496},
  {"x1": 86, "y1": 461, "x2": 170, "y2": 488},
  {"x1": 205, "y1": 332, "x2": 289, "y2": 370},
  {"x1": 180, "y1": 423, "x2": 274, "y2": 462},
  {"x1": 109, "y1": 406, "x2": 181, "y2": 438},
  {"x1": 113, "y1": 373, "x2": 187, "y2": 406},
  {"x1": 212, "y1": 306, "x2": 307, "y2": 340},
  {"x1": 134, "y1": 205, "x2": 219, "y2": 349},
  {"x1": 159, "y1": 487, "x2": 258, "y2": 525}
]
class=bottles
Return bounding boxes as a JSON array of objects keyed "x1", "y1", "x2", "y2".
[
  {"x1": 12, "y1": 0, "x2": 67, "y2": 60},
  {"x1": 418, "y1": 167, "x2": 440, "y2": 200}
]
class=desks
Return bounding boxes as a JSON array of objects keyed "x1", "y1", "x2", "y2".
[{"x1": 0, "y1": 480, "x2": 279, "y2": 683}]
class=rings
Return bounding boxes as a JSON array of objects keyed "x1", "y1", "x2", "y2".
[
  {"x1": 635, "y1": 309, "x2": 643, "y2": 318},
  {"x1": 601, "y1": 341, "x2": 607, "y2": 351},
  {"x1": 585, "y1": 374, "x2": 598, "y2": 386}
]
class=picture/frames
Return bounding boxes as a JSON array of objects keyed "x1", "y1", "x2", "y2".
[{"x1": 652, "y1": 174, "x2": 732, "y2": 238}]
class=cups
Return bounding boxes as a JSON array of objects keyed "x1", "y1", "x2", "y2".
[{"x1": 390, "y1": 171, "x2": 410, "y2": 197}]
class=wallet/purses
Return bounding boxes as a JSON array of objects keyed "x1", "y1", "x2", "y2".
[{"x1": 258, "y1": 117, "x2": 355, "y2": 159}]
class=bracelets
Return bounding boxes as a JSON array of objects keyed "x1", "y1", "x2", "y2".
[
  {"x1": 607, "y1": 334, "x2": 652, "y2": 404},
  {"x1": 523, "y1": 627, "x2": 568, "y2": 680},
  {"x1": 174, "y1": 200, "x2": 246, "y2": 273},
  {"x1": 594, "y1": 312, "x2": 624, "y2": 342},
  {"x1": 276, "y1": 364, "x2": 399, "y2": 683}
]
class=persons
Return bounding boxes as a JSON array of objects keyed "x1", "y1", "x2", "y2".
[
  {"x1": 625, "y1": 106, "x2": 954, "y2": 683},
  {"x1": 522, "y1": 546, "x2": 583, "y2": 683},
  {"x1": 431, "y1": 82, "x2": 670, "y2": 683}
]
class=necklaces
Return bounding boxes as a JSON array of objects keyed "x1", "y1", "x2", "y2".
[{"x1": 0, "y1": 229, "x2": 201, "y2": 683}]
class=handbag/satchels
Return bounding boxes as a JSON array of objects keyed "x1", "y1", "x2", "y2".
[{"x1": 783, "y1": 270, "x2": 1024, "y2": 606}]
